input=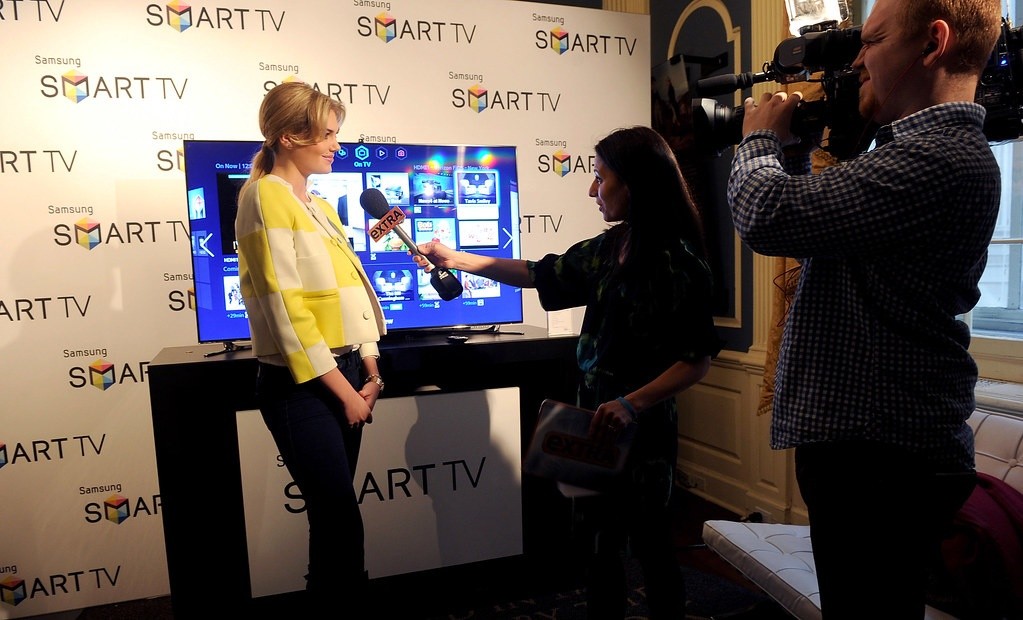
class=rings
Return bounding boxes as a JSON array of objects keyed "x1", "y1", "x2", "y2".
[{"x1": 606, "y1": 425, "x2": 615, "y2": 432}]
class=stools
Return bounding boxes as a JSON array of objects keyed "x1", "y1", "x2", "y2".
[{"x1": 556, "y1": 481, "x2": 601, "y2": 533}]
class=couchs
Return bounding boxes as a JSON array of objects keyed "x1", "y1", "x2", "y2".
[{"x1": 703, "y1": 406, "x2": 1023, "y2": 618}]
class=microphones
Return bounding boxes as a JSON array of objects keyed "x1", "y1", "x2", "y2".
[
  {"x1": 360, "y1": 188, "x2": 464, "y2": 301},
  {"x1": 696, "y1": 72, "x2": 774, "y2": 99}
]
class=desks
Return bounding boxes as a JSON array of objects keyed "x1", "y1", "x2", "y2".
[{"x1": 149, "y1": 335, "x2": 584, "y2": 620}]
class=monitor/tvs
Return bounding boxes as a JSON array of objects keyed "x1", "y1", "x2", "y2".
[{"x1": 183, "y1": 139, "x2": 525, "y2": 357}]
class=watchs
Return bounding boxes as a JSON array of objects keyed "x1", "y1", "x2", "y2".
[{"x1": 370, "y1": 373, "x2": 385, "y2": 391}]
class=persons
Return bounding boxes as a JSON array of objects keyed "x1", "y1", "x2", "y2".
[
  {"x1": 729, "y1": 0, "x2": 1001, "y2": 620},
  {"x1": 236, "y1": 82, "x2": 385, "y2": 620},
  {"x1": 406, "y1": 127, "x2": 719, "y2": 620}
]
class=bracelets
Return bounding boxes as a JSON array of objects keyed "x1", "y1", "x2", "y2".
[
  {"x1": 614, "y1": 395, "x2": 640, "y2": 424},
  {"x1": 366, "y1": 374, "x2": 382, "y2": 383}
]
